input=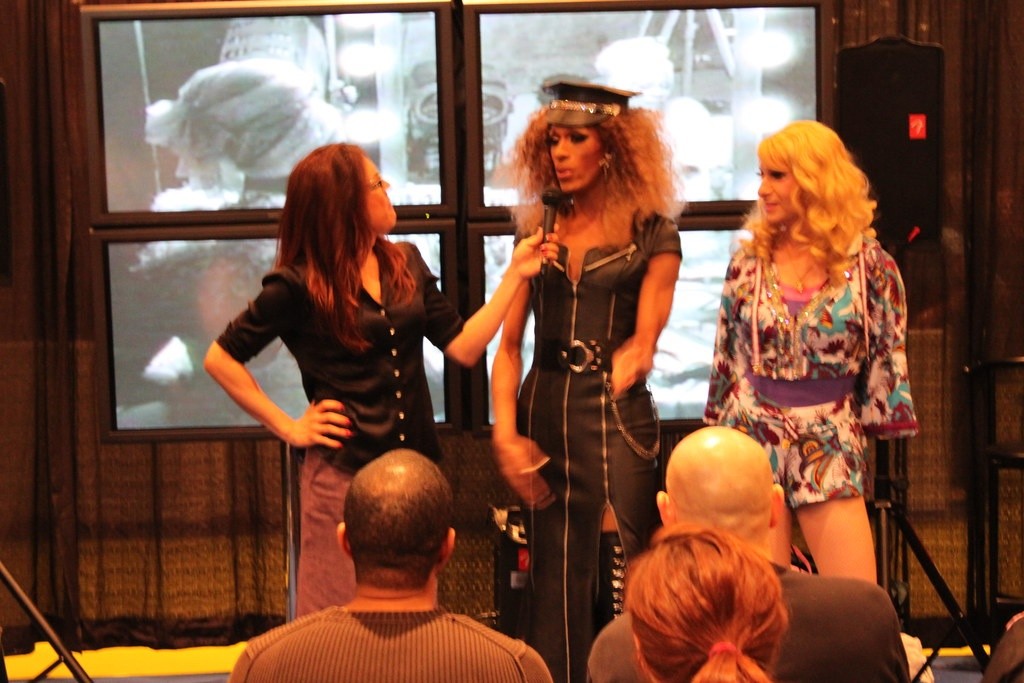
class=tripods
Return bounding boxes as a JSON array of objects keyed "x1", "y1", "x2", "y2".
[{"x1": 861, "y1": 435, "x2": 989, "y2": 668}]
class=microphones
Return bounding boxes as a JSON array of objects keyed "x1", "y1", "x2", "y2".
[{"x1": 540, "y1": 185, "x2": 563, "y2": 276}]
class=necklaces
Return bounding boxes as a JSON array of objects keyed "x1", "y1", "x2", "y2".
[{"x1": 782, "y1": 239, "x2": 815, "y2": 295}]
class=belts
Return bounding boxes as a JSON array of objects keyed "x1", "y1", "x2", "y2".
[{"x1": 532, "y1": 340, "x2": 628, "y2": 374}]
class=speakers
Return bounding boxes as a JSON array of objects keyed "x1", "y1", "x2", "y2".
[{"x1": 833, "y1": 34, "x2": 943, "y2": 244}]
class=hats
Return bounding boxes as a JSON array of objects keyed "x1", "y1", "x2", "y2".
[{"x1": 541, "y1": 73, "x2": 642, "y2": 126}]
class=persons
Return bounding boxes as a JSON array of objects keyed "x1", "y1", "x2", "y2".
[
  {"x1": 227, "y1": 447, "x2": 553, "y2": 682},
  {"x1": 587, "y1": 426, "x2": 911, "y2": 683},
  {"x1": 705, "y1": 120, "x2": 921, "y2": 584},
  {"x1": 490, "y1": 77, "x2": 682, "y2": 683},
  {"x1": 202, "y1": 142, "x2": 559, "y2": 619}
]
